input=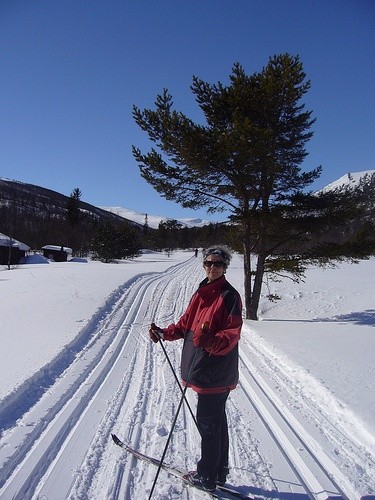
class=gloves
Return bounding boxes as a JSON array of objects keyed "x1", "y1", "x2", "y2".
[
  {"x1": 192, "y1": 327, "x2": 216, "y2": 348},
  {"x1": 148, "y1": 322, "x2": 164, "y2": 344}
]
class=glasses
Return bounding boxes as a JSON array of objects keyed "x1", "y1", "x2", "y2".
[{"x1": 202, "y1": 260, "x2": 226, "y2": 268}]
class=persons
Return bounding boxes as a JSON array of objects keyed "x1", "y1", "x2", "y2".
[
  {"x1": 149, "y1": 245, "x2": 243, "y2": 491},
  {"x1": 195, "y1": 248, "x2": 198, "y2": 257}
]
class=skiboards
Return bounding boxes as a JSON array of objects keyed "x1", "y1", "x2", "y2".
[{"x1": 110, "y1": 432, "x2": 254, "y2": 500}]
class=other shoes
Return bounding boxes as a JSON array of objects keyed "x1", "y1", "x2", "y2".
[
  {"x1": 188, "y1": 467, "x2": 229, "y2": 486},
  {"x1": 182, "y1": 472, "x2": 217, "y2": 492}
]
